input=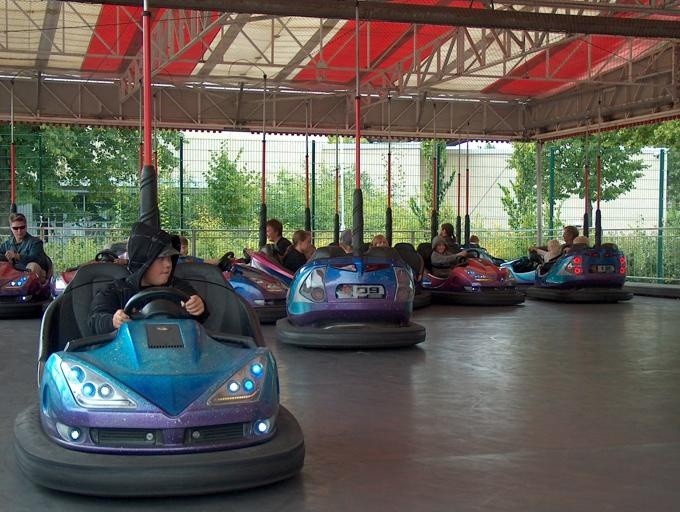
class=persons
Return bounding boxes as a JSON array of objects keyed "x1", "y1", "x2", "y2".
[
  {"x1": 468, "y1": 235, "x2": 479, "y2": 249},
  {"x1": 562, "y1": 226, "x2": 579, "y2": 249},
  {"x1": 88, "y1": 221, "x2": 211, "y2": 335},
  {"x1": 370, "y1": 235, "x2": 390, "y2": 248},
  {"x1": 430, "y1": 235, "x2": 469, "y2": 278},
  {"x1": 265, "y1": 219, "x2": 293, "y2": 266},
  {"x1": 339, "y1": 229, "x2": 353, "y2": 254},
  {"x1": 530, "y1": 240, "x2": 562, "y2": 267},
  {"x1": 438, "y1": 223, "x2": 462, "y2": 254},
  {"x1": 113, "y1": 233, "x2": 130, "y2": 265},
  {"x1": 0, "y1": 212, "x2": 48, "y2": 284},
  {"x1": 281, "y1": 230, "x2": 316, "y2": 273},
  {"x1": 175, "y1": 237, "x2": 219, "y2": 265}
]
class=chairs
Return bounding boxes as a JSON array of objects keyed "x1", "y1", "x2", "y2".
[
  {"x1": 447, "y1": 243, "x2": 463, "y2": 266},
  {"x1": 600, "y1": 243, "x2": 618, "y2": 252},
  {"x1": 364, "y1": 245, "x2": 396, "y2": 263},
  {"x1": 109, "y1": 242, "x2": 126, "y2": 260},
  {"x1": 311, "y1": 245, "x2": 347, "y2": 259},
  {"x1": 57, "y1": 261, "x2": 131, "y2": 351},
  {"x1": 417, "y1": 242, "x2": 433, "y2": 275},
  {"x1": 259, "y1": 244, "x2": 282, "y2": 264},
  {"x1": 170, "y1": 261, "x2": 244, "y2": 351},
  {"x1": 572, "y1": 243, "x2": 590, "y2": 252},
  {"x1": 394, "y1": 243, "x2": 425, "y2": 282}
]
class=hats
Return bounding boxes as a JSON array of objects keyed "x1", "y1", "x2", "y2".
[
  {"x1": 342, "y1": 230, "x2": 352, "y2": 243},
  {"x1": 157, "y1": 241, "x2": 181, "y2": 257},
  {"x1": 573, "y1": 236, "x2": 588, "y2": 244}
]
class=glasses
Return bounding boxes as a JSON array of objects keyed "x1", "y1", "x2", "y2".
[{"x1": 12, "y1": 225, "x2": 25, "y2": 230}]
